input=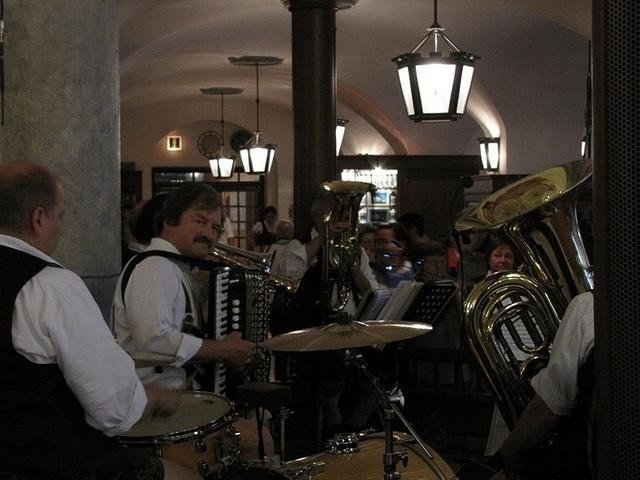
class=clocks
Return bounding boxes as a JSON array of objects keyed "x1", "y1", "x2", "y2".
[{"x1": 198, "y1": 130, "x2": 224, "y2": 158}]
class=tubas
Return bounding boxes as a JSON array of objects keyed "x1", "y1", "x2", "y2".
[
  {"x1": 320, "y1": 181, "x2": 374, "y2": 311},
  {"x1": 454, "y1": 159, "x2": 593, "y2": 432}
]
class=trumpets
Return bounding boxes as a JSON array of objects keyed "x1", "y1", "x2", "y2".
[
  {"x1": 213, "y1": 241, "x2": 276, "y2": 277},
  {"x1": 210, "y1": 243, "x2": 303, "y2": 307}
]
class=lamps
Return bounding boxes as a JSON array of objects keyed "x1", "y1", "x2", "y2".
[
  {"x1": 227, "y1": 55, "x2": 284, "y2": 175},
  {"x1": 336, "y1": 117, "x2": 350, "y2": 157},
  {"x1": 392, "y1": 0, "x2": 482, "y2": 122},
  {"x1": 476, "y1": 137, "x2": 500, "y2": 172},
  {"x1": 580, "y1": 136, "x2": 586, "y2": 157},
  {"x1": 200, "y1": 87, "x2": 243, "y2": 179}
]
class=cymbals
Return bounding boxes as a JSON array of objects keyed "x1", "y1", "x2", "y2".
[{"x1": 257, "y1": 321, "x2": 433, "y2": 351}]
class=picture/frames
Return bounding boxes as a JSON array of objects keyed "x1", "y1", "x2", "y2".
[{"x1": 166, "y1": 135, "x2": 182, "y2": 151}]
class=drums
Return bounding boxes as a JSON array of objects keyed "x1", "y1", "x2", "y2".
[
  {"x1": 208, "y1": 430, "x2": 457, "y2": 479},
  {"x1": 114, "y1": 392, "x2": 240, "y2": 480}
]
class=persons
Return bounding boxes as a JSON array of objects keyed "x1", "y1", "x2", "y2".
[
  {"x1": 106, "y1": 180, "x2": 597, "y2": 480},
  {"x1": 0, "y1": 160, "x2": 147, "y2": 480}
]
